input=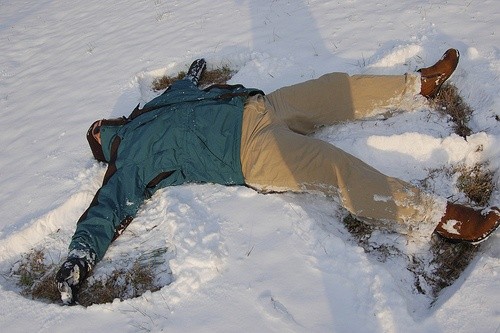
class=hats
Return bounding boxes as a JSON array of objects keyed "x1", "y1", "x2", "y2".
[{"x1": 86, "y1": 118, "x2": 108, "y2": 165}]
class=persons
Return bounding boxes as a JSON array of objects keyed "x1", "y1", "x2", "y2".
[{"x1": 56, "y1": 48, "x2": 500, "y2": 304}]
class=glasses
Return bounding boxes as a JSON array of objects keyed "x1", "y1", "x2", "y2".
[{"x1": 93, "y1": 119, "x2": 102, "y2": 136}]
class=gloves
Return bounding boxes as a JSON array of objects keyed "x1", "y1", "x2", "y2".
[
  {"x1": 51, "y1": 252, "x2": 90, "y2": 306},
  {"x1": 184, "y1": 57, "x2": 207, "y2": 82}
]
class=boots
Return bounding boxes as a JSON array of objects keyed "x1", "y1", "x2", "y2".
[
  {"x1": 434, "y1": 200, "x2": 500, "y2": 246},
  {"x1": 418, "y1": 47, "x2": 461, "y2": 99}
]
